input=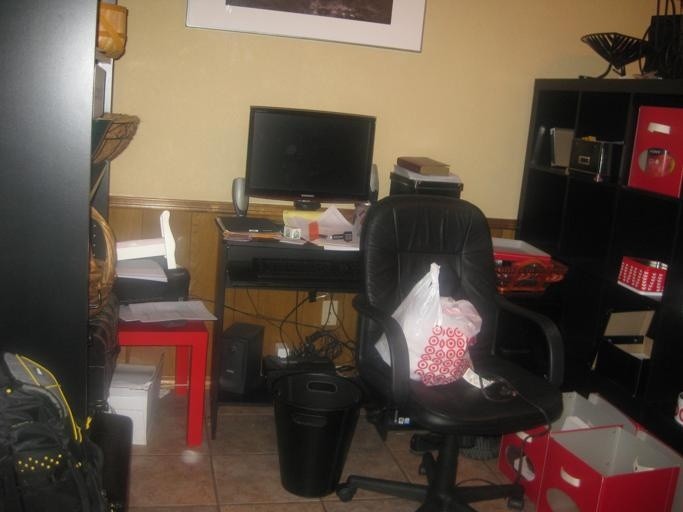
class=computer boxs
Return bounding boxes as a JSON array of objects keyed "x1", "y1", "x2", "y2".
[{"x1": 218, "y1": 321, "x2": 265, "y2": 401}]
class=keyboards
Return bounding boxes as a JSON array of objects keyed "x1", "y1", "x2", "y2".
[{"x1": 251, "y1": 258, "x2": 361, "y2": 284}]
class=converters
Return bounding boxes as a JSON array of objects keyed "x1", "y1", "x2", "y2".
[{"x1": 275, "y1": 341, "x2": 290, "y2": 358}]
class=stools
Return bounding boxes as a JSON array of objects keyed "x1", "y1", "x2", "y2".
[{"x1": 85, "y1": 416, "x2": 132, "y2": 510}]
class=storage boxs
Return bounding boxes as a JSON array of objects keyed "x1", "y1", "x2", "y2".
[
  {"x1": 108, "y1": 351, "x2": 167, "y2": 448},
  {"x1": 580, "y1": 302, "x2": 673, "y2": 413},
  {"x1": 536, "y1": 418, "x2": 679, "y2": 512},
  {"x1": 569, "y1": 137, "x2": 625, "y2": 179},
  {"x1": 498, "y1": 387, "x2": 623, "y2": 509},
  {"x1": 625, "y1": 103, "x2": 682, "y2": 199}
]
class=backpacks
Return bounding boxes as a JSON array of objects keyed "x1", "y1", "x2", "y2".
[{"x1": 0, "y1": 352, "x2": 116, "y2": 512}]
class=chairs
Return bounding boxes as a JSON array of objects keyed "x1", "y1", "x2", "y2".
[{"x1": 335, "y1": 192, "x2": 566, "y2": 511}]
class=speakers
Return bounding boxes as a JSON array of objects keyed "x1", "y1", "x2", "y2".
[
  {"x1": 369, "y1": 164, "x2": 379, "y2": 199},
  {"x1": 231, "y1": 176, "x2": 249, "y2": 217}
]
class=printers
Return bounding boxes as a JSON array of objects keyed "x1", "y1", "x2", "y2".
[{"x1": 389, "y1": 171, "x2": 464, "y2": 204}]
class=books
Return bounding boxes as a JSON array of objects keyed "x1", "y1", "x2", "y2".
[
  {"x1": 214, "y1": 215, "x2": 283, "y2": 242},
  {"x1": 393, "y1": 157, "x2": 462, "y2": 184}
]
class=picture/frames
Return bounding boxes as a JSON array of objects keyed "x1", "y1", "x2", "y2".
[{"x1": 185, "y1": 0, "x2": 427, "y2": 53}]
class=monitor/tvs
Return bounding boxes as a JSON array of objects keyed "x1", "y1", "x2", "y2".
[{"x1": 245, "y1": 105, "x2": 377, "y2": 211}]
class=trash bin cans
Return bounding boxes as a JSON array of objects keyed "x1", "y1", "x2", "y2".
[{"x1": 274, "y1": 371, "x2": 360, "y2": 498}]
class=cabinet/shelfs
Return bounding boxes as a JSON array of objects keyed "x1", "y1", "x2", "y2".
[
  {"x1": 513, "y1": 77, "x2": 683, "y2": 457},
  {"x1": 0, "y1": 1, "x2": 119, "y2": 429}
]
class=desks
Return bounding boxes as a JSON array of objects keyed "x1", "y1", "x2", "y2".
[
  {"x1": 207, "y1": 216, "x2": 551, "y2": 438},
  {"x1": 118, "y1": 319, "x2": 209, "y2": 451}
]
